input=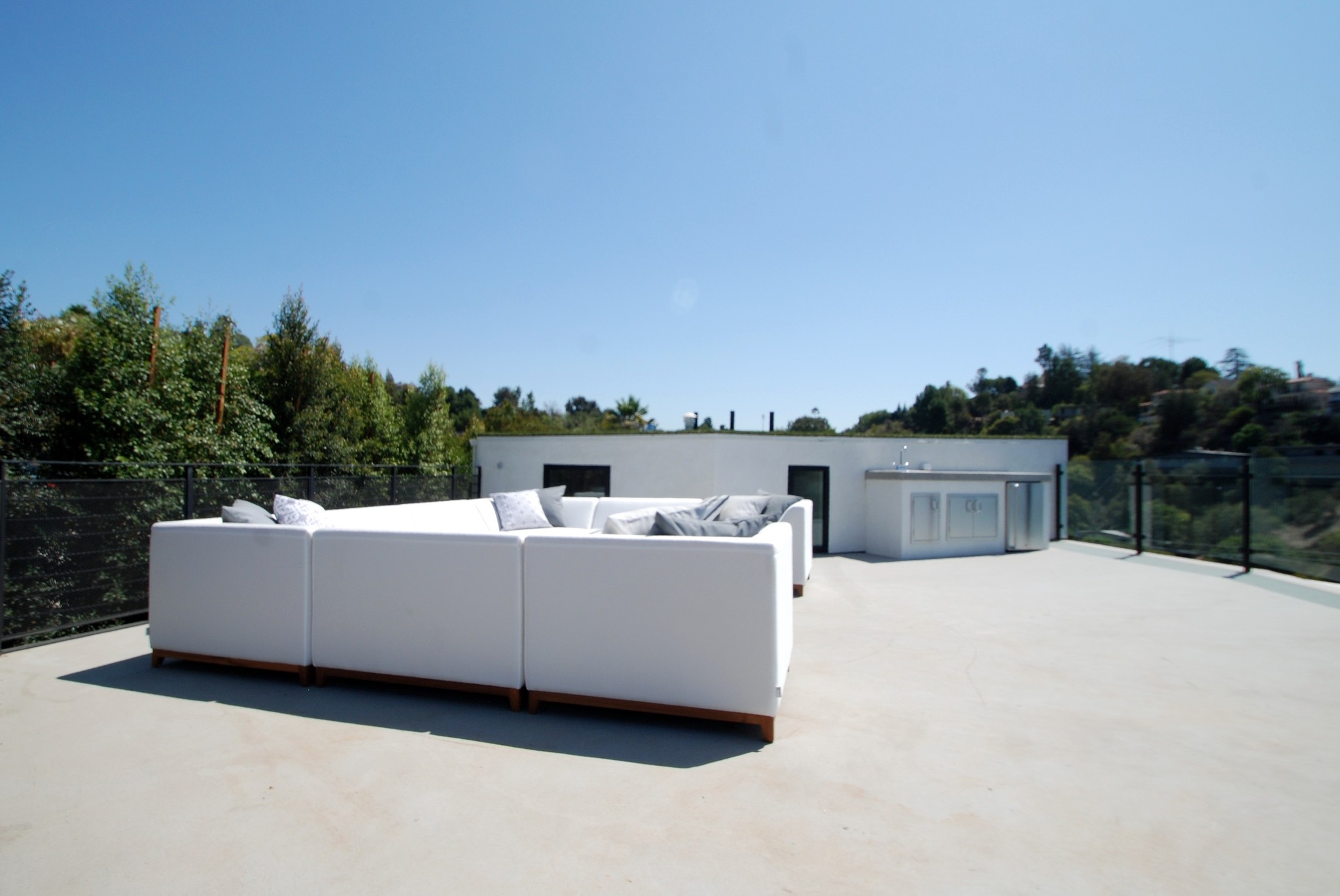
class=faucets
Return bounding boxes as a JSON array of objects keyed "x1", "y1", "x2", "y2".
[{"x1": 896, "y1": 445, "x2": 909, "y2": 470}]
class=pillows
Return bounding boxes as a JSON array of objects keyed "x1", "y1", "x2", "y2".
[{"x1": 535, "y1": 485, "x2": 569, "y2": 526}]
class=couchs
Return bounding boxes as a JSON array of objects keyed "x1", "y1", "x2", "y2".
[{"x1": 144, "y1": 490, "x2": 813, "y2": 747}]
